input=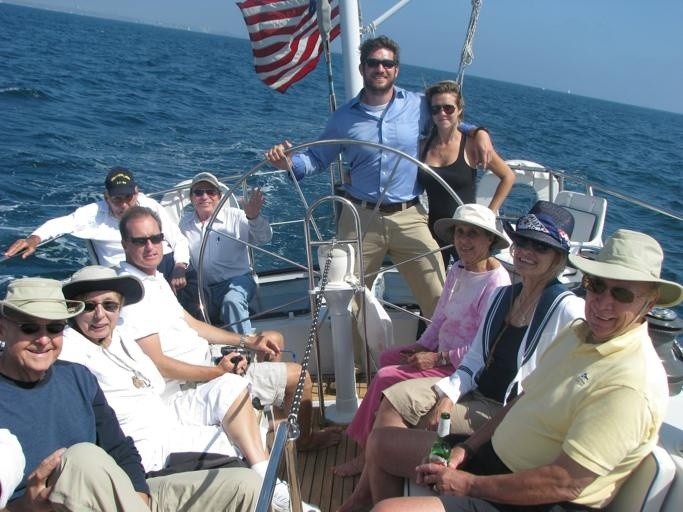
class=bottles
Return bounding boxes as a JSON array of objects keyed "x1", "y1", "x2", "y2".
[{"x1": 429, "y1": 413, "x2": 452, "y2": 475}]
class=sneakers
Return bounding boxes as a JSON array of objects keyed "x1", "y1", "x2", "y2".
[{"x1": 270, "y1": 480, "x2": 318, "y2": 512}]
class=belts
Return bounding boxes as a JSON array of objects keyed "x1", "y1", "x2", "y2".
[{"x1": 348, "y1": 195, "x2": 419, "y2": 212}]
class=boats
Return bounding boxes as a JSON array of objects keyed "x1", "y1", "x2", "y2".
[{"x1": 85, "y1": 0, "x2": 681, "y2": 508}]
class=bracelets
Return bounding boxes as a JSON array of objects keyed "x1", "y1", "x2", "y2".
[
  {"x1": 454, "y1": 443, "x2": 474, "y2": 461},
  {"x1": 239, "y1": 335, "x2": 249, "y2": 348},
  {"x1": 474, "y1": 126, "x2": 490, "y2": 138},
  {"x1": 441, "y1": 352, "x2": 446, "y2": 366}
]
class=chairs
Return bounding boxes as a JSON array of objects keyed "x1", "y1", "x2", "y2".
[
  {"x1": 604, "y1": 443, "x2": 679, "y2": 511},
  {"x1": 551, "y1": 192, "x2": 607, "y2": 289}
]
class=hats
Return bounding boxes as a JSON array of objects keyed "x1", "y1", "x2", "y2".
[
  {"x1": 105, "y1": 167, "x2": 134, "y2": 194},
  {"x1": 190, "y1": 172, "x2": 222, "y2": 198},
  {"x1": 434, "y1": 204, "x2": 510, "y2": 250},
  {"x1": 0, "y1": 277, "x2": 85, "y2": 322},
  {"x1": 568, "y1": 228, "x2": 683, "y2": 308},
  {"x1": 502, "y1": 200, "x2": 575, "y2": 253},
  {"x1": 63, "y1": 265, "x2": 145, "y2": 306}
]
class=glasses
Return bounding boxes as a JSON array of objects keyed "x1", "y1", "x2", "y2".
[
  {"x1": 582, "y1": 275, "x2": 657, "y2": 304},
  {"x1": 363, "y1": 59, "x2": 399, "y2": 68},
  {"x1": 193, "y1": 189, "x2": 215, "y2": 196},
  {"x1": 430, "y1": 105, "x2": 456, "y2": 115},
  {"x1": 3, "y1": 316, "x2": 69, "y2": 335},
  {"x1": 127, "y1": 232, "x2": 164, "y2": 245},
  {"x1": 85, "y1": 301, "x2": 119, "y2": 313},
  {"x1": 514, "y1": 235, "x2": 552, "y2": 255}
]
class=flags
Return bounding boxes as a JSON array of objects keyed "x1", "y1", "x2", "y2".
[{"x1": 235, "y1": 1, "x2": 343, "y2": 95}]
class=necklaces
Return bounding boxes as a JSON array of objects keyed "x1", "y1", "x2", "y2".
[{"x1": 517, "y1": 286, "x2": 543, "y2": 326}]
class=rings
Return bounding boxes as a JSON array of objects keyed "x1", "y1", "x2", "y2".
[
  {"x1": 432, "y1": 485, "x2": 441, "y2": 494},
  {"x1": 424, "y1": 427, "x2": 429, "y2": 430}
]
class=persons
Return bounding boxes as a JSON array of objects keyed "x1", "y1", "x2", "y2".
[
  {"x1": 1, "y1": 278, "x2": 275, "y2": 512},
  {"x1": 264, "y1": 35, "x2": 495, "y2": 394},
  {"x1": 3, "y1": 167, "x2": 213, "y2": 324},
  {"x1": 56, "y1": 266, "x2": 322, "y2": 512},
  {"x1": 116, "y1": 207, "x2": 343, "y2": 454},
  {"x1": 334, "y1": 201, "x2": 583, "y2": 512},
  {"x1": 340, "y1": 79, "x2": 515, "y2": 343},
  {"x1": 176, "y1": 173, "x2": 275, "y2": 334},
  {"x1": 323, "y1": 204, "x2": 512, "y2": 476},
  {"x1": 363, "y1": 227, "x2": 683, "y2": 512}
]
